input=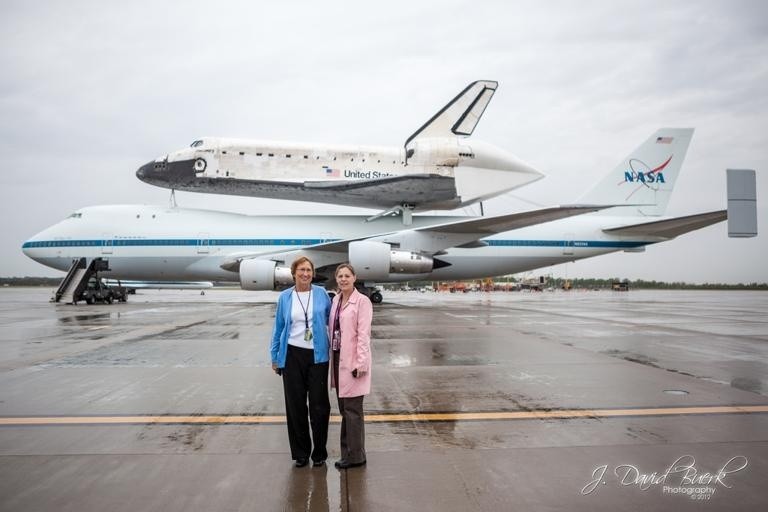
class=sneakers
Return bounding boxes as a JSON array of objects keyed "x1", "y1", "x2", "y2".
[
  {"x1": 296, "y1": 457, "x2": 309, "y2": 467},
  {"x1": 313, "y1": 459, "x2": 325, "y2": 466},
  {"x1": 335, "y1": 459, "x2": 366, "y2": 468}
]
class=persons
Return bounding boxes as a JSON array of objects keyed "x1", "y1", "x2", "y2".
[
  {"x1": 328, "y1": 264, "x2": 373, "y2": 468},
  {"x1": 270, "y1": 256, "x2": 332, "y2": 467}
]
